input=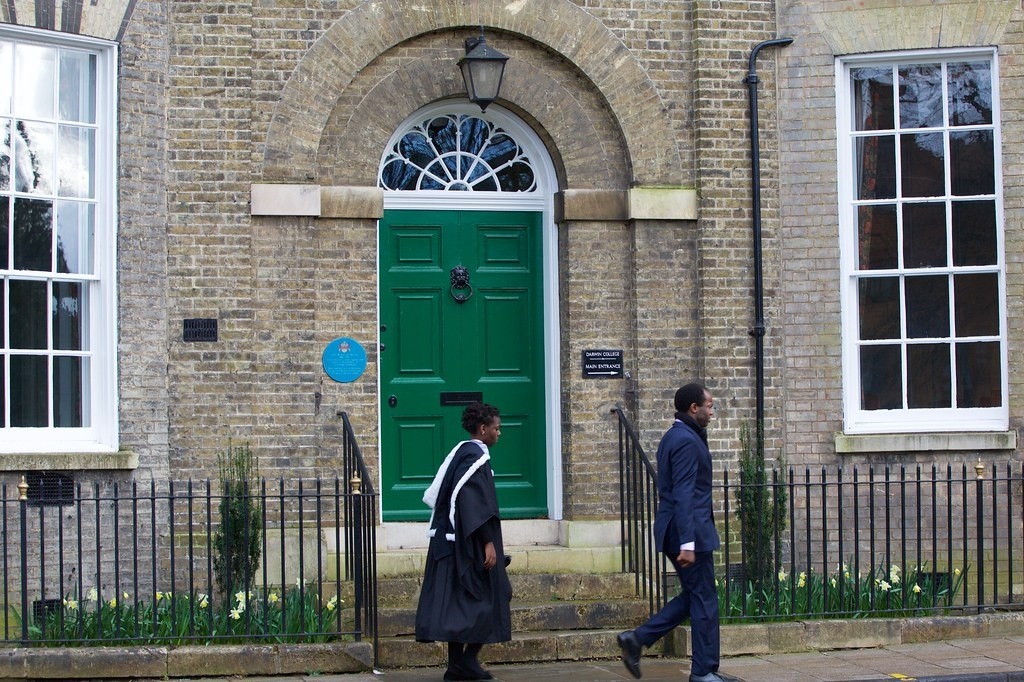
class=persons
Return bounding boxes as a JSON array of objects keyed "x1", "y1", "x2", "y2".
[
  {"x1": 614, "y1": 382, "x2": 725, "y2": 682},
  {"x1": 414, "y1": 405, "x2": 512, "y2": 681}
]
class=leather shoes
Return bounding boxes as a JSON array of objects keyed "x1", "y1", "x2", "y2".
[
  {"x1": 618, "y1": 630, "x2": 641, "y2": 679},
  {"x1": 689, "y1": 672, "x2": 737, "y2": 682}
]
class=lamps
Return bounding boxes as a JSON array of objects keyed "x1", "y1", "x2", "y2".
[{"x1": 454, "y1": 22, "x2": 511, "y2": 115}]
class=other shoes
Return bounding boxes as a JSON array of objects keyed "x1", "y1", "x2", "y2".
[{"x1": 443, "y1": 653, "x2": 493, "y2": 682}]
